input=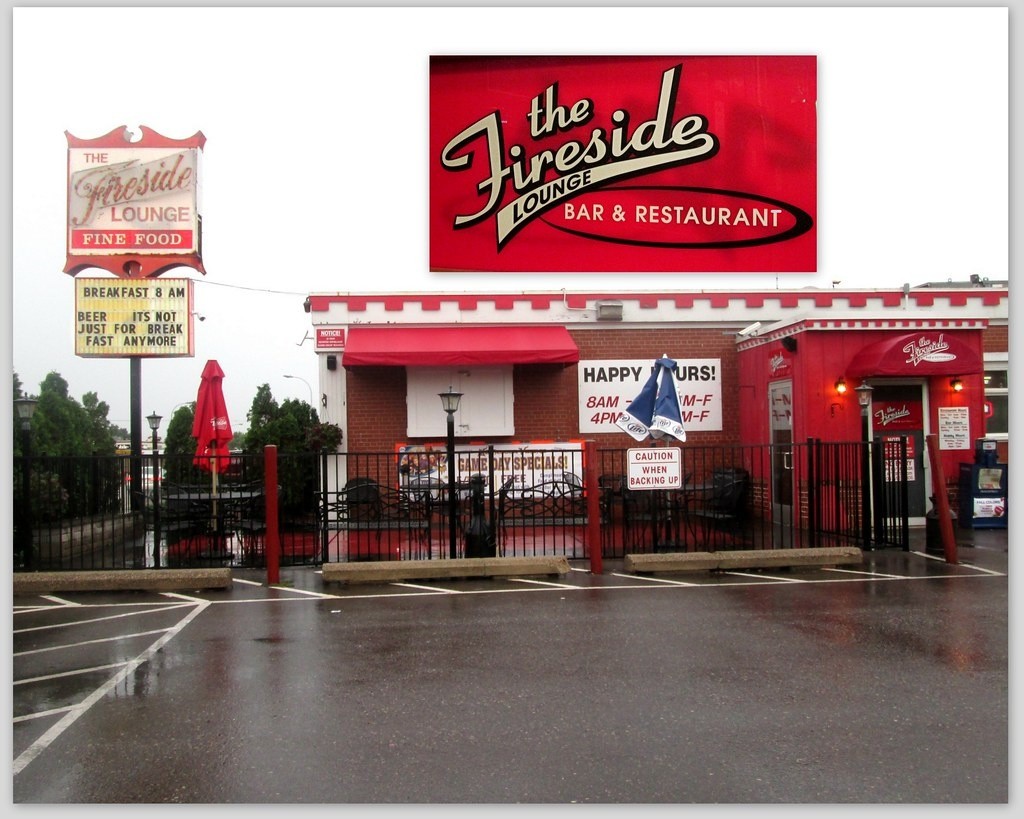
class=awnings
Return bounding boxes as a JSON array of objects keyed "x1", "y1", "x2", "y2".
[
  {"x1": 342, "y1": 325, "x2": 580, "y2": 372},
  {"x1": 848, "y1": 330, "x2": 989, "y2": 378}
]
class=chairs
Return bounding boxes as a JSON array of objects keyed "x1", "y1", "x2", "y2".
[
  {"x1": 464, "y1": 477, "x2": 515, "y2": 545},
  {"x1": 599, "y1": 473, "x2": 632, "y2": 525},
  {"x1": 341, "y1": 476, "x2": 383, "y2": 542},
  {"x1": 130, "y1": 473, "x2": 283, "y2": 562}
]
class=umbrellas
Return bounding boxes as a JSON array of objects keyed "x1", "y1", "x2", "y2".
[
  {"x1": 194, "y1": 360, "x2": 234, "y2": 533},
  {"x1": 615, "y1": 352, "x2": 689, "y2": 532}
]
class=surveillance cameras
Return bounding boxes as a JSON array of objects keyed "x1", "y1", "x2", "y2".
[{"x1": 738, "y1": 321, "x2": 761, "y2": 337}]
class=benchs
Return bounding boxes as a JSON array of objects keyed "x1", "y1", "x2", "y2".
[
  {"x1": 685, "y1": 467, "x2": 750, "y2": 533},
  {"x1": 312, "y1": 483, "x2": 432, "y2": 562}
]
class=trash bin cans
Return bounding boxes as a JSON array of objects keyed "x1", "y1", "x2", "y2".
[{"x1": 710, "y1": 467, "x2": 749, "y2": 515}]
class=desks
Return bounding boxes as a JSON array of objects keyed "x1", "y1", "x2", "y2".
[
  {"x1": 399, "y1": 483, "x2": 472, "y2": 540},
  {"x1": 162, "y1": 492, "x2": 261, "y2": 559},
  {"x1": 657, "y1": 484, "x2": 720, "y2": 550}
]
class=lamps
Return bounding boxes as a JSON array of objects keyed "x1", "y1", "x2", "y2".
[
  {"x1": 782, "y1": 336, "x2": 799, "y2": 351},
  {"x1": 835, "y1": 377, "x2": 847, "y2": 395},
  {"x1": 594, "y1": 299, "x2": 623, "y2": 323},
  {"x1": 950, "y1": 376, "x2": 963, "y2": 392}
]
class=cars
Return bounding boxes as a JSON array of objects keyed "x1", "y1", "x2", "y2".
[{"x1": 125, "y1": 466, "x2": 167, "y2": 491}]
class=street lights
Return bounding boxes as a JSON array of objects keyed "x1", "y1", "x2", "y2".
[
  {"x1": 439, "y1": 387, "x2": 466, "y2": 559},
  {"x1": 282, "y1": 374, "x2": 313, "y2": 412},
  {"x1": 148, "y1": 411, "x2": 163, "y2": 567},
  {"x1": 171, "y1": 402, "x2": 194, "y2": 421}
]
